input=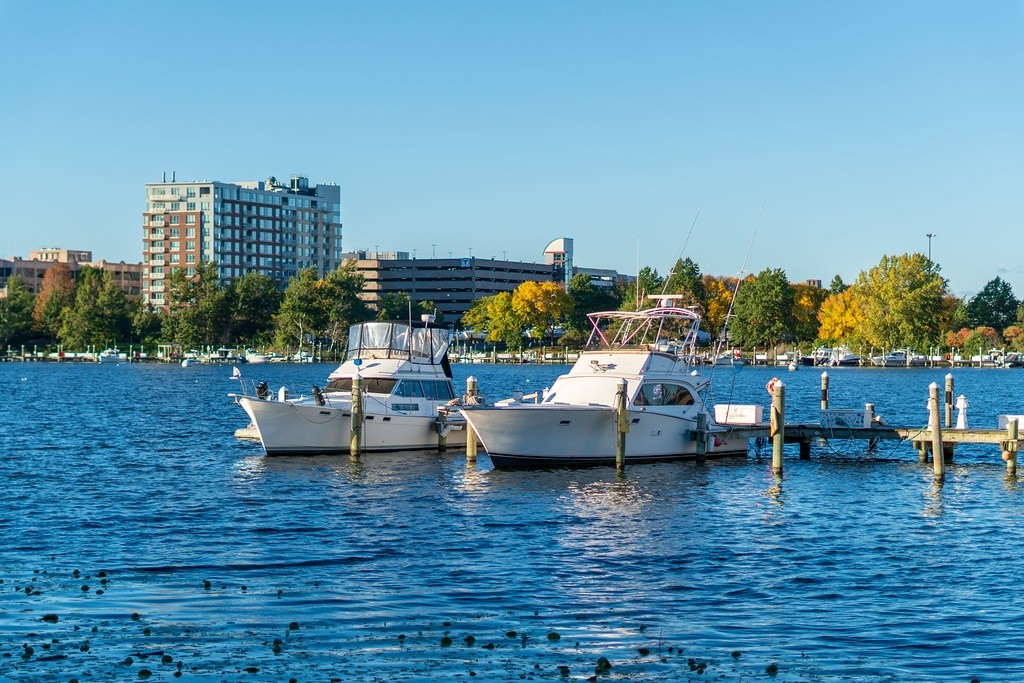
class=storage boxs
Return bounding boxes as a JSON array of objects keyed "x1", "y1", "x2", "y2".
[
  {"x1": 997, "y1": 414, "x2": 1024, "y2": 429},
  {"x1": 821, "y1": 410, "x2": 873, "y2": 428},
  {"x1": 713, "y1": 404, "x2": 765, "y2": 424}
]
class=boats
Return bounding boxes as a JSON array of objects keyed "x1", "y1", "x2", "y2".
[
  {"x1": 228, "y1": 319, "x2": 485, "y2": 456},
  {"x1": 709, "y1": 339, "x2": 1024, "y2": 368},
  {"x1": 435, "y1": 294, "x2": 764, "y2": 473},
  {"x1": 97, "y1": 335, "x2": 272, "y2": 364}
]
